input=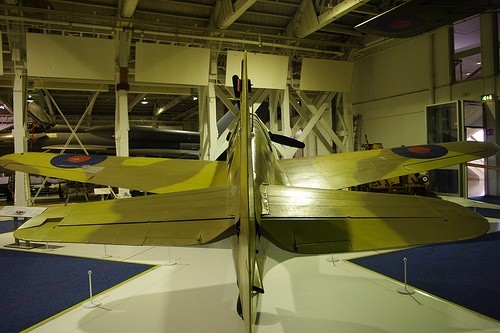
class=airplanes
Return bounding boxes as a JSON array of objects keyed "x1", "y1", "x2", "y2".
[{"x1": 0, "y1": 52, "x2": 500, "y2": 332}]
